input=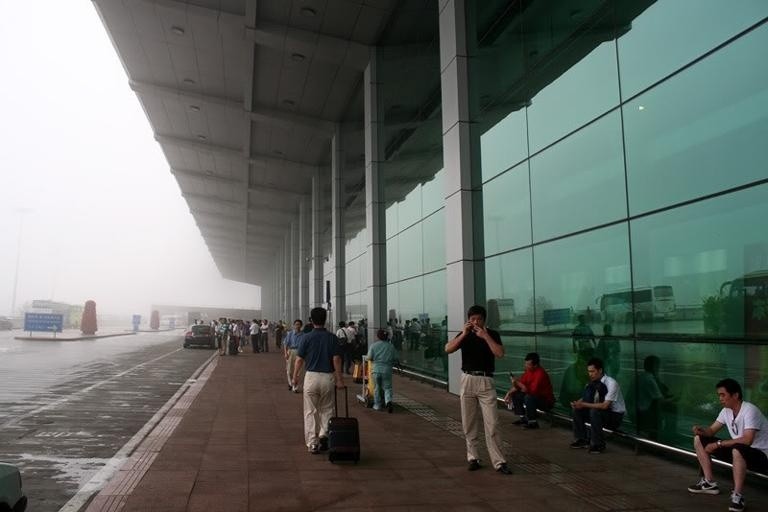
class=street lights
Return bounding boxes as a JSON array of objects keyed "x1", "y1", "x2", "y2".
[{"x1": 10, "y1": 206, "x2": 31, "y2": 317}]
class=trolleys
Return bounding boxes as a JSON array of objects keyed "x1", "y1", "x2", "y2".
[{"x1": 353, "y1": 354, "x2": 375, "y2": 408}]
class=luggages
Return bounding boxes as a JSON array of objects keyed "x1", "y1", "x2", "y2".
[{"x1": 327, "y1": 383, "x2": 361, "y2": 468}]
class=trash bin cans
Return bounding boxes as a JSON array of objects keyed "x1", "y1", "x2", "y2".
[{"x1": 365, "y1": 360, "x2": 384, "y2": 407}]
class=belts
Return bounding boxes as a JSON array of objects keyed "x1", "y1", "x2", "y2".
[{"x1": 462, "y1": 367, "x2": 494, "y2": 379}]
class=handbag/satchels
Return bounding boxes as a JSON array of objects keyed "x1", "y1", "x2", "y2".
[{"x1": 336, "y1": 328, "x2": 348, "y2": 346}]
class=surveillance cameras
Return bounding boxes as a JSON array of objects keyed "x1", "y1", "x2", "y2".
[
  {"x1": 326, "y1": 257, "x2": 329, "y2": 261},
  {"x1": 306, "y1": 257, "x2": 310, "y2": 262}
]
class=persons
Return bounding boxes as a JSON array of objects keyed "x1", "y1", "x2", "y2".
[
  {"x1": 503, "y1": 352, "x2": 556, "y2": 429},
  {"x1": 570, "y1": 357, "x2": 628, "y2": 454},
  {"x1": 358, "y1": 315, "x2": 448, "y2": 357},
  {"x1": 444, "y1": 304, "x2": 512, "y2": 475},
  {"x1": 191, "y1": 317, "x2": 358, "y2": 393},
  {"x1": 291, "y1": 307, "x2": 344, "y2": 453},
  {"x1": 686, "y1": 378, "x2": 768, "y2": 512},
  {"x1": 558, "y1": 314, "x2": 682, "y2": 433},
  {"x1": 366, "y1": 329, "x2": 400, "y2": 414}
]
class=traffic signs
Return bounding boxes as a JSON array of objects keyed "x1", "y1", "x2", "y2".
[
  {"x1": 23, "y1": 313, "x2": 64, "y2": 334},
  {"x1": 543, "y1": 307, "x2": 571, "y2": 327}
]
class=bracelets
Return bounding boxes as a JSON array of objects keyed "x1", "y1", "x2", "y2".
[{"x1": 716, "y1": 440, "x2": 721, "y2": 448}]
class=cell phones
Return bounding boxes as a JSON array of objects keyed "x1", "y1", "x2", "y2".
[{"x1": 510, "y1": 373, "x2": 513, "y2": 377}]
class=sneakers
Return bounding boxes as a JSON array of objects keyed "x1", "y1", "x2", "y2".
[
  {"x1": 287, "y1": 383, "x2": 300, "y2": 393},
  {"x1": 569, "y1": 440, "x2": 607, "y2": 455},
  {"x1": 216, "y1": 347, "x2": 271, "y2": 354},
  {"x1": 727, "y1": 488, "x2": 746, "y2": 512},
  {"x1": 687, "y1": 477, "x2": 721, "y2": 495},
  {"x1": 307, "y1": 435, "x2": 329, "y2": 454},
  {"x1": 372, "y1": 400, "x2": 394, "y2": 413}
]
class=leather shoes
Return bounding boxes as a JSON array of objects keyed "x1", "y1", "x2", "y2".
[
  {"x1": 511, "y1": 416, "x2": 541, "y2": 430},
  {"x1": 496, "y1": 465, "x2": 514, "y2": 475},
  {"x1": 468, "y1": 460, "x2": 482, "y2": 472}
]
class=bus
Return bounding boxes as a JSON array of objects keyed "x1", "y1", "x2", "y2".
[
  {"x1": 484, "y1": 295, "x2": 517, "y2": 323},
  {"x1": 716, "y1": 266, "x2": 768, "y2": 339},
  {"x1": 32, "y1": 299, "x2": 73, "y2": 329},
  {"x1": 594, "y1": 280, "x2": 677, "y2": 324},
  {"x1": 69, "y1": 304, "x2": 86, "y2": 329}
]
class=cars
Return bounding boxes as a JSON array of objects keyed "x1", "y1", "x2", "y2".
[
  {"x1": 0, "y1": 314, "x2": 14, "y2": 333},
  {"x1": 0, "y1": 459, "x2": 29, "y2": 512},
  {"x1": 178, "y1": 322, "x2": 216, "y2": 352}
]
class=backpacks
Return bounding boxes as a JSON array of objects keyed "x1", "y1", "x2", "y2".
[{"x1": 582, "y1": 380, "x2": 608, "y2": 424}]
